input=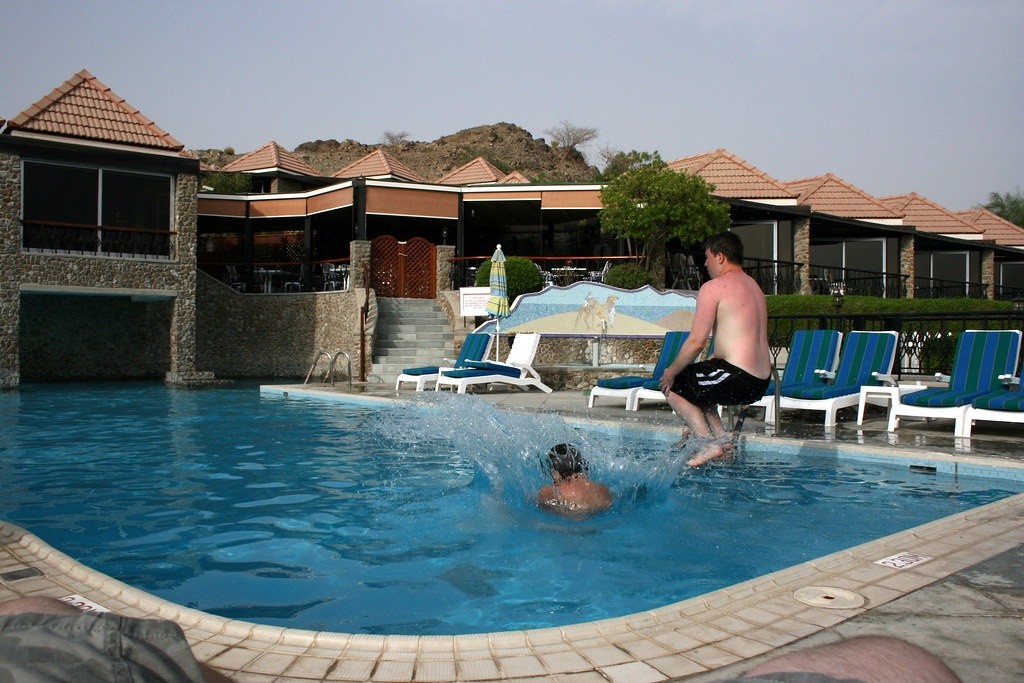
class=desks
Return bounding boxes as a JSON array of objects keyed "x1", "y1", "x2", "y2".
[
  {"x1": 553, "y1": 267, "x2": 587, "y2": 272},
  {"x1": 330, "y1": 268, "x2": 350, "y2": 290},
  {"x1": 254, "y1": 270, "x2": 300, "y2": 294},
  {"x1": 809, "y1": 277, "x2": 825, "y2": 291}
]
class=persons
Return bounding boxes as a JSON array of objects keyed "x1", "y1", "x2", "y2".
[
  {"x1": 537, "y1": 440, "x2": 609, "y2": 520},
  {"x1": 675, "y1": 422, "x2": 733, "y2": 464},
  {"x1": 657, "y1": 231, "x2": 772, "y2": 464}
]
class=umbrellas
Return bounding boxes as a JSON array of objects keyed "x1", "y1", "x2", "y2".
[{"x1": 484, "y1": 243, "x2": 510, "y2": 362}]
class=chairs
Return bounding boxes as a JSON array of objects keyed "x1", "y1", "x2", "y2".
[
  {"x1": 396, "y1": 331, "x2": 495, "y2": 391},
  {"x1": 583, "y1": 260, "x2": 613, "y2": 283},
  {"x1": 585, "y1": 331, "x2": 702, "y2": 412},
  {"x1": 226, "y1": 264, "x2": 249, "y2": 293},
  {"x1": 717, "y1": 329, "x2": 843, "y2": 422},
  {"x1": 963, "y1": 363, "x2": 1024, "y2": 438},
  {"x1": 320, "y1": 263, "x2": 344, "y2": 291},
  {"x1": 824, "y1": 268, "x2": 843, "y2": 295},
  {"x1": 433, "y1": 333, "x2": 553, "y2": 396},
  {"x1": 634, "y1": 337, "x2": 713, "y2": 414},
  {"x1": 285, "y1": 264, "x2": 315, "y2": 292},
  {"x1": 889, "y1": 328, "x2": 1023, "y2": 437},
  {"x1": 771, "y1": 330, "x2": 900, "y2": 427},
  {"x1": 465, "y1": 266, "x2": 478, "y2": 287},
  {"x1": 534, "y1": 263, "x2": 556, "y2": 288}
]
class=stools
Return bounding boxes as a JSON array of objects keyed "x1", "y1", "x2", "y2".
[{"x1": 860, "y1": 385, "x2": 928, "y2": 427}]
